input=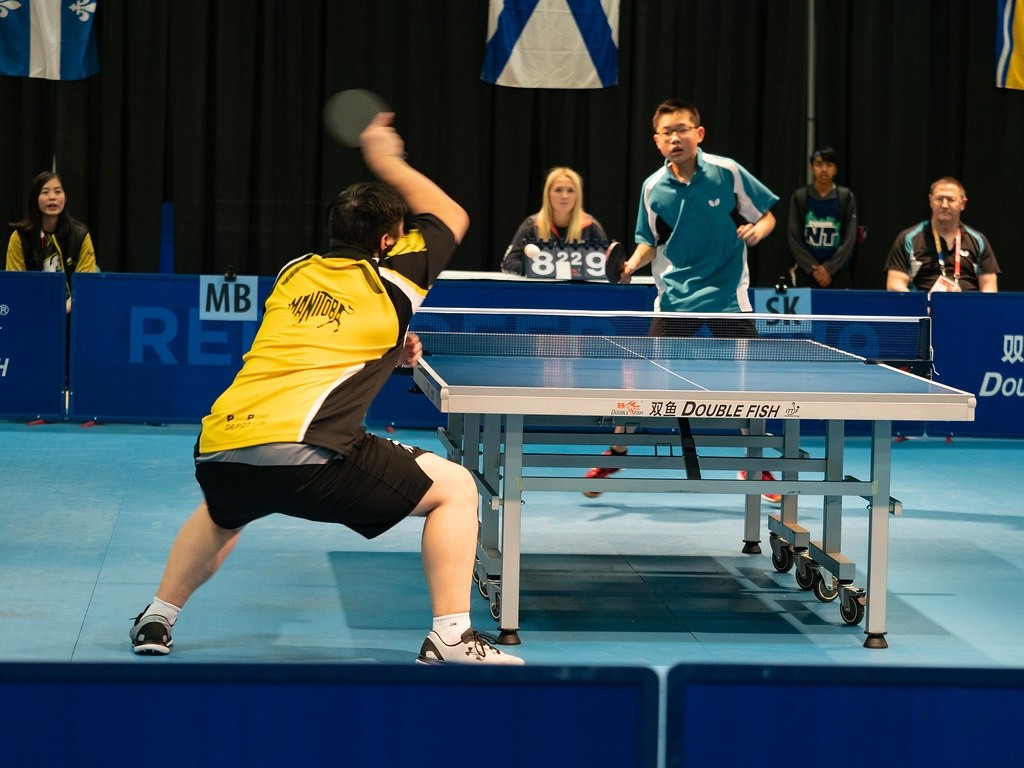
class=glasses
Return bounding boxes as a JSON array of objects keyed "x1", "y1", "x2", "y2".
[{"x1": 656, "y1": 125, "x2": 698, "y2": 138}]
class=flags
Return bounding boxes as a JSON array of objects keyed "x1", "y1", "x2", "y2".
[
  {"x1": 991, "y1": 0, "x2": 1023, "y2": 88},
  {"x1": 0, "y1": 0, "x2": 97, "y2": 80},
  {"x1": 479, "y1": 0, "x2": 620, "y2": 89}
]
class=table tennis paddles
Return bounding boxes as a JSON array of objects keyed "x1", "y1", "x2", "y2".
[
  {"x1": 325, "y1": 90, "x2": 386, "y2": 145},
  {"x1": 603, "y1": 241, "x2": 627, "y2": 283}
]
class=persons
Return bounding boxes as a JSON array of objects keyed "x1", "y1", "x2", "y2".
[
  {"x1": 5, "y1": 170, "x2": 98, "y2": 273},
  {"x1": 128, "y1": 112, "x2": 527, "y2": 666},
  {"x1": 788, "y1": 149, "x2": 859, "y2": 289},
  {"x1": 888, "y1": 177, "x2": 1000, "y2": 293},
  {"x1": 503, "y1": 168, "x2": 610, "y2": 275},
  {"x1": 581, "y1": 101, "x2": 786, "y2": 502}
]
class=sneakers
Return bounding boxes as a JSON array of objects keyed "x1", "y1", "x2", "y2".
[
  {"x1": 129, "y1": 604, "x2": 173, "y2": 655},
  {"x1": 581, "y1": 450, "x2": 625, "y2": 498},
  {"x1": 415, "y1": 627, "x2": 525, "y2": 665},
  {"x1": 737, "y1": 470, "x2": 781, "y2": 503}
]
